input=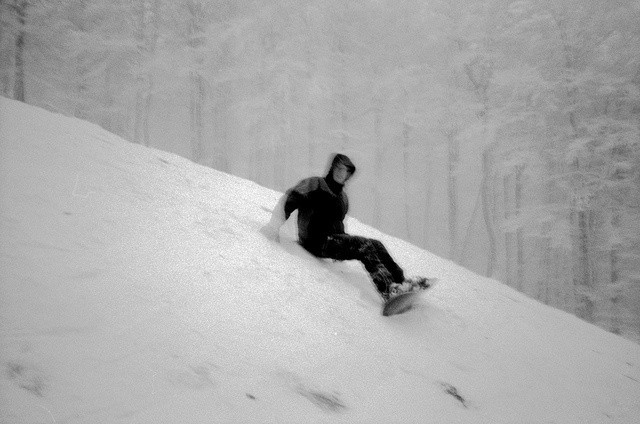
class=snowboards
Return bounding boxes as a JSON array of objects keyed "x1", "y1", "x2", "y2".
[{"x1": 383, "y1": 276, "x2": 430, "y2": 316}]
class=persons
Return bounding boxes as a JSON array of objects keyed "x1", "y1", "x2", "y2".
[{"x1": 260, "y1": 153, "x2": 430, "y2": 316}]
